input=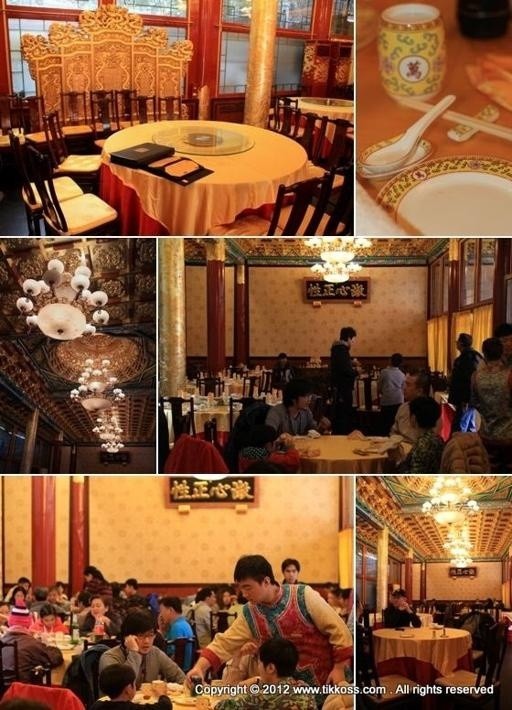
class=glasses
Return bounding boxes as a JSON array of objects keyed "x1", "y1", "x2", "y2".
[{"x1": 138, "y1": 631, "x2": 156, "y2": 640}]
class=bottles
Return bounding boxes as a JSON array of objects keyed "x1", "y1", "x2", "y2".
[
  {"x1": 94, "y1": 614, "x2": 104, "y2": 642},
  {"x1": 70, "y1": 613, "x2": 80, "y2": 645}
]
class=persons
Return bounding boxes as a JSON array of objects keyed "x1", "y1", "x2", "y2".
[
  {"x1": 185, "y1": 555, "x2": 354, "y2": 710},
  {"x1": 324, "y1": 581, "x2": 354, "y2": 635},
  {"x1": 281, "y1": 558, "x2": 306, "y2": 584},
  {"x1": 0, "y1": 564, "x2": 247, "y2": 710},
  {"x1": 224, "y1": 321, "x2": 512, "y2": 474},
  {"x1": 214, "y1": 636, "x2": 320, "y2": 710},
  {"x1": 384, "y1": 589, "x2": 421, "y2": 628}
]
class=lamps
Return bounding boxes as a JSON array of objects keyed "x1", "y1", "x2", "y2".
[
  {"x1": 13, "y1": 257, "x2": 110, "y2": 341},
  {"x1": 102, "y1": 438, "x2": 124, "y2": 454},
  {"x1": 310, "y1": 260, "x2": 364, "y2": 285},
  {"x1": 421, "y1": 477, "x2": 482, "y2": 569},
  {"x1": 70, "y1": 357, "x2": 125, "y2": 413},
  {"x1": 303, "y1": 238, "x2": 374, "y2": 266},
  {"x1": 93, "y1": 414, "x2": 124, "y2": 440}
]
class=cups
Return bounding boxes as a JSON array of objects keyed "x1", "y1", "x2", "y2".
[
  {"x1": 152, "y1": 680, "x2": 164, "y2": 689},
  {"x1": 374, "y1": 3, "x2": 449, "y2": 100}
]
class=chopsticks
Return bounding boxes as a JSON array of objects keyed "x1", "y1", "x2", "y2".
[{"x1": 387, "y1": 88, "x2": 511, "y2": 151}]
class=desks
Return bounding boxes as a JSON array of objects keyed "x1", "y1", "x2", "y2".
[
  {"x1": 91, "y1": 681, "x2": 237, "y2": 710},
  {"x1": 29, "y1": 630, "x2": 93, "y2": 684},
  {"x1": 291, "y1": 363, "x2": 328, "y2": 379},
  {"x1": 284, "y1": 433, "x2": 414, "y2": 473},
  {"x1": 433, "y1": 390, "x2": 449, "y2": 404}
]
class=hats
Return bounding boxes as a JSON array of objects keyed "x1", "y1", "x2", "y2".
[{"x1": 7, "y1": 599, "x2": 33, "y2": 627}]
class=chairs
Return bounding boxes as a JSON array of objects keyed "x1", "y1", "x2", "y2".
[
  {"x1": 430, "y1": 370, "x2": 444, "y2": 380},
  {"x1": 29, "y1": 665, "x2": 52, "y2": 687},
  {"x1": 357, "y1": 599, "x2": 508, "y2": 709},
  {"x1": 0, "y1": 639, "x2": 19, "y2": 690},
  {"x1": 163, "y1": 635, "x2": 197, "y2": 673},
  {"x1": 83, "y1": 638, "x2": 120, "y2": 650},
  {"x1": 355, "y1": 376, "x2": 383, "y2": 416},
  {"x1": 160, "y1": 368, "x2": 282, "y2": 444},
  {"x1": 209, "y1": 610, "x2": 236, "y2": 648}
]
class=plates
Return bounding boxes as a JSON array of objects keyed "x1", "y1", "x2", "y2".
[
  {"x1": 58, "y1": 643, "x2": 74, "y2": 651},
  {"x1": 401, "y1": 633, "x2": 414, "y2": 638},
  {"x1": 372, "y1": 153, "x2": 511, "y2": 236},
  {"x1": 173, "y1": 696, "x2": 197, "y2": 706},
  {"x1": 357, "y1": 139, "x2": 435, "y2": 181}
]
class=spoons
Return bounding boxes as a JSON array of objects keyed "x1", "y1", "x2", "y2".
[{"x1": 358, "y1": 94, "x2": 456, "y2": 173}]
